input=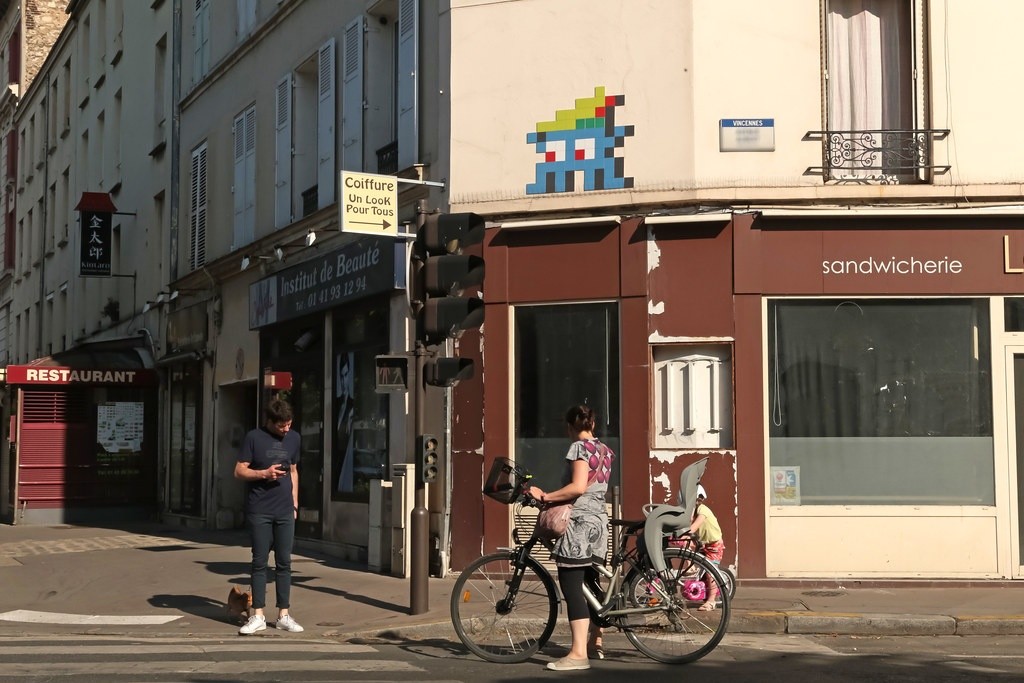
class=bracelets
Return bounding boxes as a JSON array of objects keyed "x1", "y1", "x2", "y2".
[{"x1": 541, "y1": 492, "x2": 547, "y2": 504}]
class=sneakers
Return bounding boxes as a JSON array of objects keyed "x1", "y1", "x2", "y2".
[
  {"x1": 276, "y1": 613, "x2": 304, "y2": 632},
  {"x1": 238, "y1": 613, "x2": 267, "y2": 633}
]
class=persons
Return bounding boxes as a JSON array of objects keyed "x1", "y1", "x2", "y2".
[
  {"x1": 233, "y1": 397, "x2": 305, "y2": 637},
  {"x1": 670, "y1": 482, "x2": 725, "y2": 611},
  {"x1": 522, "y1": 402, "x2": 616, "y2": 671}
]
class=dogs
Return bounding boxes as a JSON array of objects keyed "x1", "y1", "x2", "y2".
[{"x1": 225, "y1": 585, "x2": 252, "y2": 627}]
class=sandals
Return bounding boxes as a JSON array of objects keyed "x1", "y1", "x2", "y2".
[
  {"x1": 682, "y1": 565, "x2": 699, "y2": 577},
  {"x1": 699, "y1": 600, "x2": 718, "y2": 612}
]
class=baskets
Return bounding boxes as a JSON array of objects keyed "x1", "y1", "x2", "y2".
[{"x1": 480, "y1": 455, "x2": 533, "y2": 506}]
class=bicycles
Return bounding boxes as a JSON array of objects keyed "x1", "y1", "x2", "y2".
[
  {"x1": 449, "y1": 456, "x2": 732, "y2": 666},
  {"x1": 629, "y1": 534, "x2": 737, "y2": 615}
]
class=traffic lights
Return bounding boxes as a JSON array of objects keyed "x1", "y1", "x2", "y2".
[{"x1": 411, "y1": 211, "x2": 488, "y2": 389}]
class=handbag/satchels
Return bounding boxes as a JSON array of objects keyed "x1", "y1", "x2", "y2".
[{"x1": 535, "y1": 499, "x2": 574, "y2": 537}]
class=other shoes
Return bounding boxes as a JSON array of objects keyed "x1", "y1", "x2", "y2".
[
  {"x1": 547, "y1": 656, "x2": 591, "y2": 672},
  {"x1": 586, "y1": 648, "x2": 605, "y2": 660}
]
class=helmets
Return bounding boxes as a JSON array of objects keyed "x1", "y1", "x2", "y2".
[{"x1": 696, "y1": 484, "x2": 708, "y2": 500}]
